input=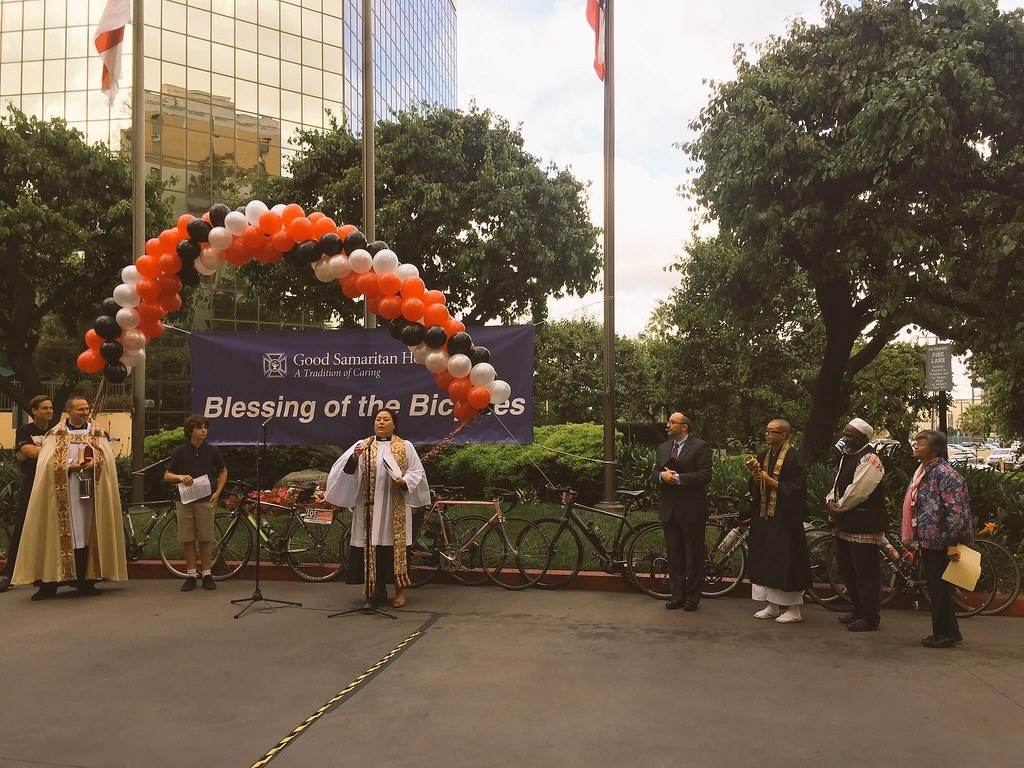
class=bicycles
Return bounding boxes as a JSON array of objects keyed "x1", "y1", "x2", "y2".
[
  {"x1": 339, "y1": 485, "x2": 550, "y2": 591},
  {"x1": 117, "y1": 480, "x2": 223, "y2": 578},
  {"x1": 626, "y1": 492, "x2": 1021, "y2": 618},
  {"x1": 515, "y1": 484, "x2": 662, "y2": 594},
  {"x1": 196, "y1": 478, "x2": 351, "y2": 582}
]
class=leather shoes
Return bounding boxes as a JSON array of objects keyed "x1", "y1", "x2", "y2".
[
  {"x1": 666, "y1": 600, "x2": 683, "y2": 610},
  {"x1": 683, "y1": 601, "x2": 698, "y2": 611}
]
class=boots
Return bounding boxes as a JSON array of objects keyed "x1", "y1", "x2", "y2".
[
  {"x1": 31, "y1": 583, "x2": 57, "y2": 600},
  {"x1": 69, "y1": 548, "x2": 100, "y2": 595}
]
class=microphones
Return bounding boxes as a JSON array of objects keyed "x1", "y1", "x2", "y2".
[
  {"x1": 261, "y1": 417, "x2": 273, "y2": 427},
  {"x1": 365, "y1": 436, "x2": 375, "y2": 448}
]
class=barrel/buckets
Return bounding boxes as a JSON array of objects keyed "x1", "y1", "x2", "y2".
[{"x1": 79, "y1": 468, "x2": 91, "y2": 499}]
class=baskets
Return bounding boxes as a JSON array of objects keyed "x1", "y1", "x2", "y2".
[{"x1": 221, "y1": 481, "x2": 246, "y2": 512}]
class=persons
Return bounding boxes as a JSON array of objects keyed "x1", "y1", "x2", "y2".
[
  {"x1": 326, "y1": 408, "x2": 430, "y2": 608},
  {"x1": 902, "y1": 430, "x2": 973, "y2": 646},
  {"x1": 0, "y1": 395, "x2": 54, "y2": 592},
  {"x1": 653, "y1": 412, "x2": 713, "y2": 611},
  {"x1": 10, "y1": 395, "x2": 128, "y2": 601},
  {"x1": 747, "y1": 419, "x2": 808, "y2": 623},
  {"x1": 163, "y1": 414, "x2": 228, "y2": 592},
  {"x1": 826, "y1": 418, "x2": 885, "y2": 632}
]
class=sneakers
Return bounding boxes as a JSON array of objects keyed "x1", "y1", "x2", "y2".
[
  {"x1": 181, "y1": 577, "x2": 196, "y2": 591},
  {"x1": 203, "y1": 575, "x2": 216, "y2": 590}
]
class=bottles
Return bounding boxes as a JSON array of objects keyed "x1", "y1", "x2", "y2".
[
  {"x1": 259, "y1": 517, "x2": 275, "y2": 537},
  {"x1": 444, "y1": 518, "x2": 457, "y2": 543},
  {"x1": 718, "y1": 526, "x2": 741, "y2": 554},
  {"x1": 881, "y1": 536, "x2": 899, "y2": 561},
  {"x1": 141, "y1": 510, "x2": 160, "y2": 535},
  {"x1": 458, "y1": 527, "x2": 476, "y2": 545},
  {"x1": 587, "y1": 520, "x2": 605, "y2": 543}
]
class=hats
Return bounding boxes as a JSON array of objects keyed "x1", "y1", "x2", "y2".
[{"x1": 848, "y1": 418, "x2": 874, "y2": 441}]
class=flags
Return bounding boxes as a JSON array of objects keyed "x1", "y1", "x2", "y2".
[
  {"x1": 586, "y1": 0, "x2": 605, "y2": 82},
  {"x1": 94, "y1": 0, "x2": 131, "y2": 107}
]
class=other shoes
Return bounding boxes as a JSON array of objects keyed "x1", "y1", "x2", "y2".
[
  {"x1": 0, "y1": 575, "x2": 11, "y2": 592},
  {"x1": 927, "y1": 632, "x2": 963, "y2": 642},
  {"x1": 838, "y1": 612, "x2": 859, "y2": 622},
  {"x1": 923, "y1": 635, "x2": 956, "y2": 648},
  {"x1": 775, "y1": 612, "x2": 802, "y2": 623},
  {"x1": 754, "y1": 605, "x2": 780, "y2": 618},
  {"x1": 393, "y1": 599, "x2": 405, "y2": 608},
  {"x1": 847, "y1": 615, "x2": 881, "y2": 631}
]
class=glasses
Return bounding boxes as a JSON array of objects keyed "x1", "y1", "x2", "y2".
[
  {"x1": 667, "y1": 420, "x2": 687, "y2": 425},
  {"x1": 764, "y1": 429, "x2": 782, "y2": 434}
]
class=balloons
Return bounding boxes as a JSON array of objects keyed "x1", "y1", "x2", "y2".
[{"x1": 76, "y1": 198, "x2": 511, "y2": 421}]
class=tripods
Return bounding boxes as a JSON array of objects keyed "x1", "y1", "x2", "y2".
[
  {"x1": 327, "y1": 447, "x2": 397, "y2": 619},
  {"x1": 230, "y1": 425, "x2": 302, "y2": 619}
]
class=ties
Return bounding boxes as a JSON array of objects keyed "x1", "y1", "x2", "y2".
[{"x1": 671, "y1": 443, "x2": 679, "y2": 460}]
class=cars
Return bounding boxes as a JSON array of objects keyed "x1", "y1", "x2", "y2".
[{"x1": 948, "y1": 441, "x2": 1024, "y2": 472}]
